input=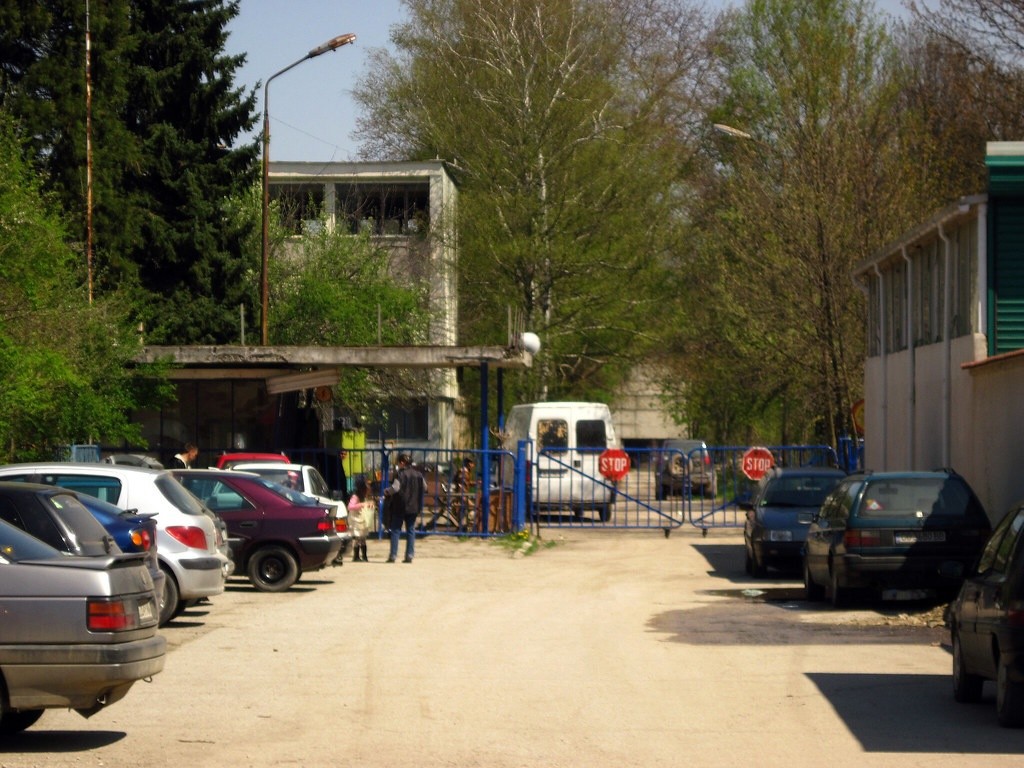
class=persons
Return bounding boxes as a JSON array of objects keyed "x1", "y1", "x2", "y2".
[
  {"x1": 330, "y1": 449, "x2": 350, "y2": 507},
  {"x1": 449, "y1": 458, "x2": 482, "y2": 533},
  {"x1": 347, "y1": 474, "x2": 374, "y2": 562},
  {"x1": 163, "y1": 442, "x2": 199, "y2": 470},
  {"x1": 383, "y1": 454, "x2": 427, "y2": 563}
]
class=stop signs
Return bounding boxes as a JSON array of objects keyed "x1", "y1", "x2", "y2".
[
  {"x1": 597, "y1": 449, "x2": 630, "y2": 480},
  {"x1": 742, "y1": 447, "x2": 774, "y2": 481}
]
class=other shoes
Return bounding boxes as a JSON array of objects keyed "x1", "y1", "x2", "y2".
[
  {"x1": 402, "y1": 557, "x2": 412, "y2": 563},
  {"x1": 386, "y1": 558, "x2": 396, "y2": 563}
]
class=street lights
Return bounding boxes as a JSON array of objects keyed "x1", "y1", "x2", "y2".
[
  {"x1": 259, "y1": 31, "x2": 360, "y2": 345},
  {"x1": 711, "y1": 123, "x2": 851, "y2": 467}
]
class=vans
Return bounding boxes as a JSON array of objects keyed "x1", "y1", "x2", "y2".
[{"x1": 490, "y1": 401, "x2": 617, "y2": 522}]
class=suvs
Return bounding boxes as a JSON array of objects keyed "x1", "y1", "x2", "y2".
[
  {"x1": 652, "y1": 439, "x2": 718, "y2": 501},
  {"x1": 0, "y1": 461, "x2": 229, "y2": 630}
]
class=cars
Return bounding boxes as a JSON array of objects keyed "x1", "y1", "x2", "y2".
[
  {"x1": 0, "y1": 482, "x2": 122, "y2": 561},
  {"x1": 66, "y1": 489, "x2": 166, "y2": 610},
  {"x1": 804, "y1": 466, "x2": 993, "y2": 609},
  {"x1": 744, "y1": 466, "x2": 848, "y2": 579},
  {"x1": 161, "y1": 450, "x2": 350, "y2": 592},
  {"x1": 935, "y1": 506, "x2": 1024, "y2": 730},
  {"x1": 0, "y1": 518, "x2": 167, "y2": 747}
]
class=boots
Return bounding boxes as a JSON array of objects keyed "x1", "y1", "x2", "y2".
[
  {"x1": 360, "y1": 543, "x2": 369, "y2": 562},
  {"x1": 352, "y1": 545, "x2": 362, "y2": 561}
]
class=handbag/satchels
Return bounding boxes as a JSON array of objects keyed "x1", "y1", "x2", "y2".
[
  {"x1": 382, "y1": 489, "x2": 404, "y2": 530},
  {"x1": 359, "y1": 503, "x2": 379, "y2": 532}
]
